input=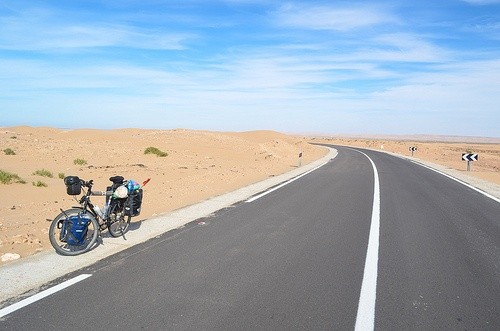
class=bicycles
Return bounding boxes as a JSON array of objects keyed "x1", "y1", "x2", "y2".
[{"x1": 47, "y1": 175, "x2": 142, "y2": 255}]
